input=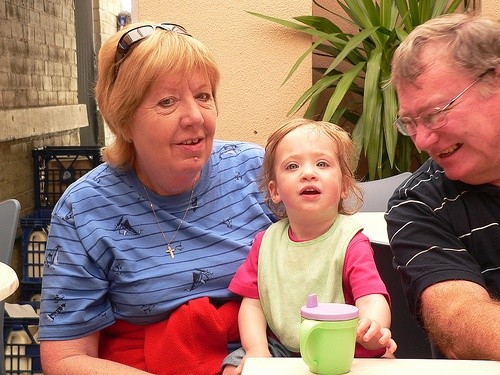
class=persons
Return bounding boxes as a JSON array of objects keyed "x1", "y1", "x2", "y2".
[
  {"x1": 384, "y1": 12, "x2": 500, "y2": 362},
  {"x1": 221, "y1": 118, "x2": 396, "y2": 375},
  {"x1": 38, "y1": 21, "x2": 288, "y2": 375}
]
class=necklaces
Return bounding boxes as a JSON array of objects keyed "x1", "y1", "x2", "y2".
[{"x1": 144, "y1": 178, "x2": 196, "y2": 258}]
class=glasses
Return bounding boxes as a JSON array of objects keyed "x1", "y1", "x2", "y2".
[
  {"x1": 391, "y1": 68, "x2": 495, "y2": 136},
  {"x1": 114, "y1": 22, "x2": 194, "y2": 83}
]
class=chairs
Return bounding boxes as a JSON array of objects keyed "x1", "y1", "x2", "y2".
[
  {"x1": 340, "y1": 171, "x2": 441, "y2": 359},
  {"x1": 0, "y1": 199, "x2": 21, "y2": 375}
]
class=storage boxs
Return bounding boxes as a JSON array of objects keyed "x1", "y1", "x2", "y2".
[
  {"x1": 20, "y1": 208, "x2": 53, "y2": 301},
  {"x1": 4, "y1": 301, "x2": 44, "y2": 375},
  {"x1": 31, "y1": 146, "x2": 105, "y2": 210}
]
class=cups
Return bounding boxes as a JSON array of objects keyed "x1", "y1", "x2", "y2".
[{"x1": 298, "y1": 294, "x2": 359, "y2": 375}]
